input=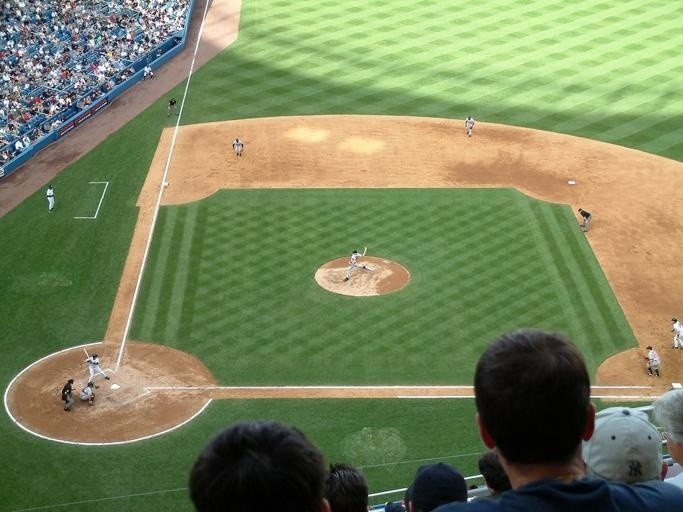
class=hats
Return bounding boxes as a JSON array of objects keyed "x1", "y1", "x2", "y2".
[
  {"x1": 410, "y1": 462, "x2": 468, "y2": 508},
  {"x1": 582, "y1": 406, "x2": 663, "y2": 483}
]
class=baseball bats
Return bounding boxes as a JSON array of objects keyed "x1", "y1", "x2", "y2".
[{"x1": 82, "y1": 348, "x2": 90, "y2": 358}]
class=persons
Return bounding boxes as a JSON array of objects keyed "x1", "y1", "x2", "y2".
[
  {"x1": 233, "y1": 138, "x2": 243, "y2": 157},
  {"x1": 84, "y1": 353, "x2": 110, "y2": 383},
  {"x1": 343, "y1": 250, "x2": 365, "y2": 281},
  {"x1": 578, "y1": 209, "x2": 592, "y2": 232},
  {"x1": 61, "y1": 379, "x2": 76, "y2": 411},
  {"x1": 0, "y1": 0, "x2": 191, "y2": 167},
  {"x1": 465, "y1": 116, "x2": 475, "y2": 137},
  {"x1": 190, "y1": 318, "x2": 683, "y2": 512},
  {"x1": 47, "y1": 185, "x2": 55, "y2": 213},
  {"x1": 80, "y1": 381, "x2": 95, "y2": 406}
]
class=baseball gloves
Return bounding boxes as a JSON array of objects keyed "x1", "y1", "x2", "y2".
[
  {"x1": 644, "y1": 356, "x2": 648, "y2": 361},
  {"x1": 240, "y1": 148, "x2": 243, "y2": 151}
]
